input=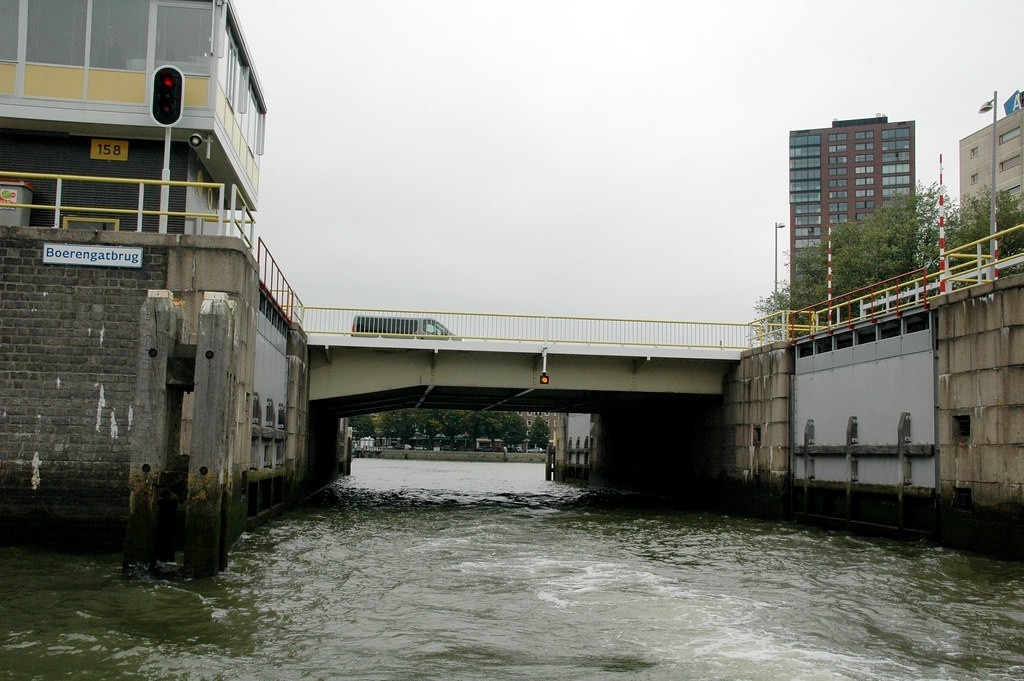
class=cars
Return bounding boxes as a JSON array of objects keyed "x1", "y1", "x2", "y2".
[{"x1": 349, "y1": 436, "x2": 547, "y2": 454}]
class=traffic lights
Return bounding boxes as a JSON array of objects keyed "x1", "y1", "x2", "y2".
[
  {"x1": 149, "y1": 64, "x2": 185, "y2": 127},
  {"x1": 539, "y1": 375, "x2": 549, "y2": 384}
]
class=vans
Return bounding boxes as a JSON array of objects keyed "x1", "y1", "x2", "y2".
[{"x1": 351, "y1": 314, "x2": 461, "y2": 342}]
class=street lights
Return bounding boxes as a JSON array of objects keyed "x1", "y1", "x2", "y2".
[
  {"x1": 978, "y1": 90, "x2": 997, "y2": 282},
  {"x1": 774, "y1": 222, "x2": 786, "y2": 343}
]
